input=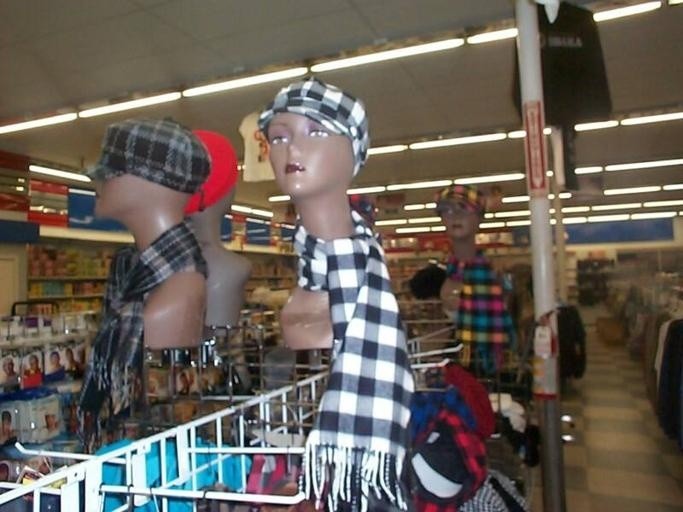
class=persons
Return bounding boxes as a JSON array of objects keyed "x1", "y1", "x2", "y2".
[
  {"x1": 74, "y1": 117, "x2": 208, "y2": 347},
  {"x1": 181, "y1": 126, "x2": 255, "y2": 327},
  {"x1": 253, "y1": 74, "x2": 386, "y2": 351},
  {"x1": 432, "y1": 178, "x2": 506, "y2": 318},
  {"x1": 0, "y1": 349, "x2": 196, "y2": 485}
]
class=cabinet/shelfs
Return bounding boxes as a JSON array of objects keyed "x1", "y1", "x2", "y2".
[
  {"x1": 18, "y1": 243, "x2": 108, "y2": 321},
  {"x1": 240, "y1": 250, "x2": 299, "y2": 305}
]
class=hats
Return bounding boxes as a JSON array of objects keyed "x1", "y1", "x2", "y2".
[
  {"x1": 255, "y1": 74, "x2": 373, "y2": 180},
  {"x1": 431, "y1": 182, "x2": 488, "y2": 221},
  {"x1": 86, "y1": 111, "x2": 213, "y2": 197}
]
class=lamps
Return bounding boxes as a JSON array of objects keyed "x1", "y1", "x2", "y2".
[
  {"x1": 359, "y1": 101, "x2": 682, "y2": 158},
  {"x1": 1, "y1": 1, "x2": 679, "y2": 137}
]
